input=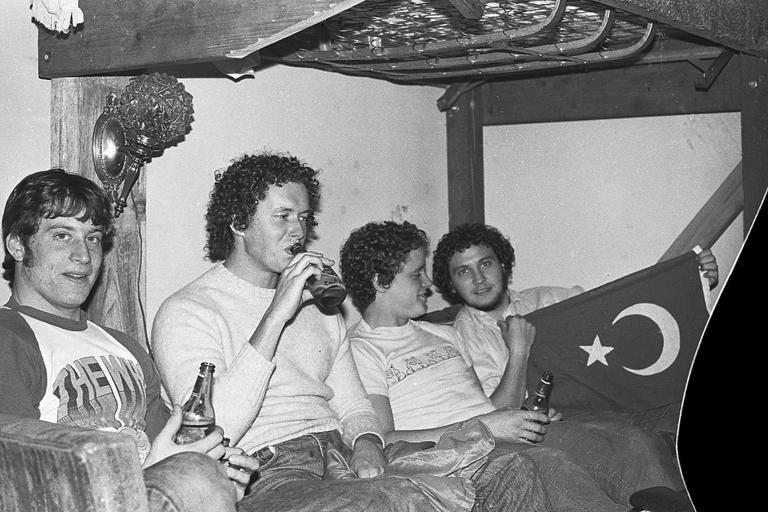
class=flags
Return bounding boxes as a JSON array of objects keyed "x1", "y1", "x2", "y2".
[{"x1": 526, "y1": 245, "x2": 715, "y2": 410}]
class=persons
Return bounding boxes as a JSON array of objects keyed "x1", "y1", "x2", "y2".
[
  {"x1": 153, "y1": 154, "x2": 552, "y2": 512},
  {"x1": 432, "y1": 223, "x2": 719, "y2": 404},
  {"x1": 340, "y1": 219, "x2": 675, "y2": 511},
  {"x1": 0, "y1": 170, "x2": 390, "y2": 511}
]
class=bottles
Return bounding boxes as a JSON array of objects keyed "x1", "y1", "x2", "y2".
[
  {"x1": 173, "y1": 362, "x2": 216, "y2": 447},
  {"x1": 291, "y1": 241, "x2": 346, "y2": 311},
  {"x1": 518, "y1": 370, "x2": 554, "y2": 443}
]
